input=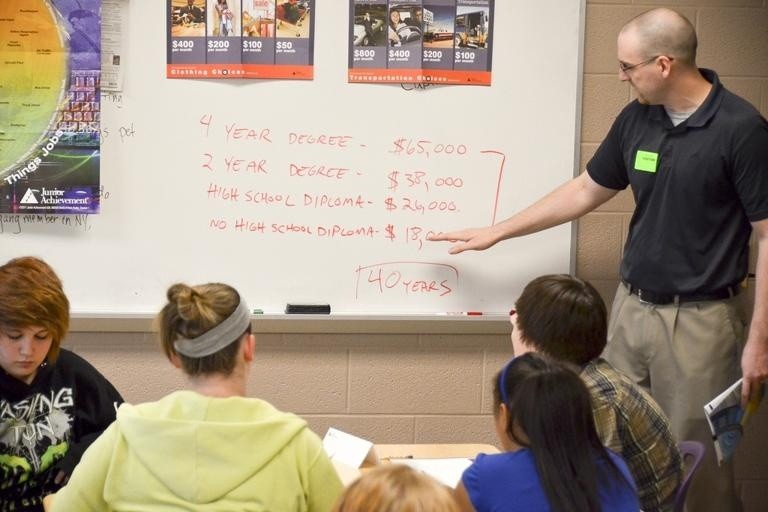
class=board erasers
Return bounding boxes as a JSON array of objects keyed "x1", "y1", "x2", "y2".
[{"x1": 285, "y1": 305, "x2": 330, "y2": 314}]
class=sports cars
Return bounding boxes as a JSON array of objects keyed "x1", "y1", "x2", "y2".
[{"x1": 354, "y1": 15, "x2": 384, "y2": 45}]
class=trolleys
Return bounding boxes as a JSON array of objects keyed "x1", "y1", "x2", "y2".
[{"x1": 276, "y1": 4, "x2": 308, "y2": 37}]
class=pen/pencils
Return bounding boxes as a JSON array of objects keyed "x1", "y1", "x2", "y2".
[
  {"x1": 380, "y1": 456, "x2": 413, "y2": 460},
  {"x1": 510, "y1": 310, "x2": 516, "y2": 315},
  {"x1": 446, "y1": 312, "x2": 482, "y2": 315}
]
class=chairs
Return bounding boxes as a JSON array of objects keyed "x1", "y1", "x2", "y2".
[{"x1": 672, "y1": 440, "x2": 706, "y2": 511}]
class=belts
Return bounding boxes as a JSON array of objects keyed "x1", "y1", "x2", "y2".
[{"x1": 622, "y1": 280, "x2": 738, "y2": 305}]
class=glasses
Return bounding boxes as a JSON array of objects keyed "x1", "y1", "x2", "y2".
[{"x1": 620, "y1": 56, "x2": 658, "y2": 71}]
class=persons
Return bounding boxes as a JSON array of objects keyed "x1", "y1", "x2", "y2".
[
  {"x1": 388, "y1": 8, "x2": 411, "y2": 47},
  {"x1": 1, "y1": 255, "x2": 124, "y2": 512},
  {"x1": 178, "y1": 0, "x2": 201, "y2": 24},
  {"x1": 37, "y1": 281, "x2": 338, "y2": 512},
  {"x1": 288, "y1": 0, "x2": 300, "y2": 23},
  {"x1": 423, "y1": 7, "x2": 768, "y2": 509},
  {"x1": 362, "y1": 12, "x2": 376, "y2": 48},
  {"x1": 214, "y1": 0, "x2": 231, "y2": 38},
  {"x1": 511, "y1": 273, "x2": 688, "y2": 511},
  {"x1": 442, "y1": 350, "x2": 643, "y2": 511},
  {"x1": 334, "y1": 461, "x2": 459, "y2": 512},
  {"x1": 409, "y1": 6, "x2": 423, "y2": 31}
]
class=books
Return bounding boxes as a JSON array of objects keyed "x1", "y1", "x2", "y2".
[{"x1": 701, "y1": 378, "x2": 744, "y2": 470}]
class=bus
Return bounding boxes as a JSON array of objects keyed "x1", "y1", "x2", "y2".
[{"x1": 455, "y1": 12, "x2": 488, "y2": 48}]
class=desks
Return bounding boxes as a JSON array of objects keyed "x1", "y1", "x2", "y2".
[{"x1": 358, "y1": 442, "x2": 501, "y2": 478}]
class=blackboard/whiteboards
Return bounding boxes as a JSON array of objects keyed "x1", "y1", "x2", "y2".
[{"x1": 0, "y1": 0, "x2": 587, "y2": 333}]
